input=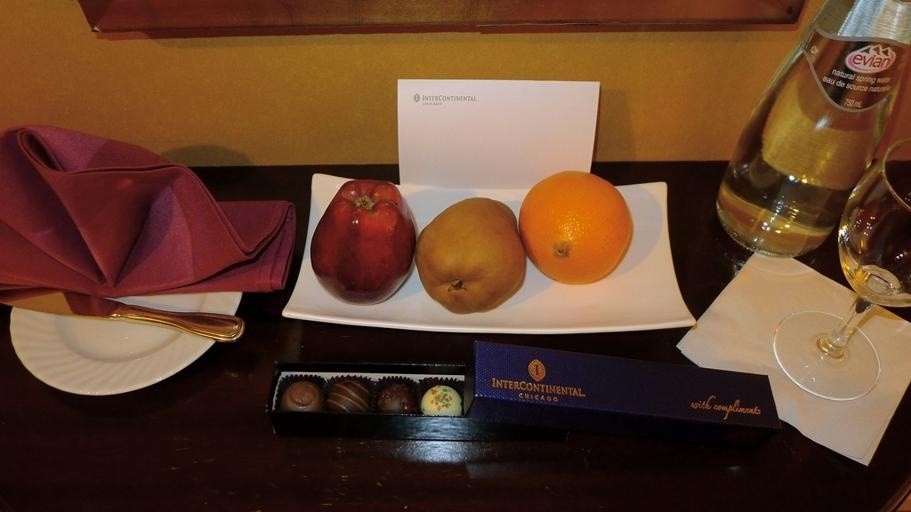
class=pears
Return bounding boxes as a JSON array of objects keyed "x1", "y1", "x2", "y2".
[{"x1": 414, "y1": 197, "x2": 530, "y2": 316}]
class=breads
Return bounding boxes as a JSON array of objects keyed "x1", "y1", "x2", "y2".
[{"x1": 281, "y1": 378, "x2": 464, "y2": 419}]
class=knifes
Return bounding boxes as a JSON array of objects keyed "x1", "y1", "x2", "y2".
[{"x1": 0, "y1": 287, "x2": 247, "y2": 343}]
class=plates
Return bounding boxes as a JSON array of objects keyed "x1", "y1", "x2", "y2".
[
  {"x1": 282, "y1": 173, "x2": 698, "y2": 334},
  {"x1": 10, "y1": 291, "x2": 242, "y2": 397}
]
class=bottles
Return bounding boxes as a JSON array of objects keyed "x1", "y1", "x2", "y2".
[{"x1": 717, "y1": 2, "x2": 911, "y2": 260}]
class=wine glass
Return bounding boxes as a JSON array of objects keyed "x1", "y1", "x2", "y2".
[{"x1": 773, "y1": 135, "x2": 910, "y2": 400}]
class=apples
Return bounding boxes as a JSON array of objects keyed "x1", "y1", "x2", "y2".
[{"x1": 309, "y1": 175, "x2": 419, "y2": 307}]
